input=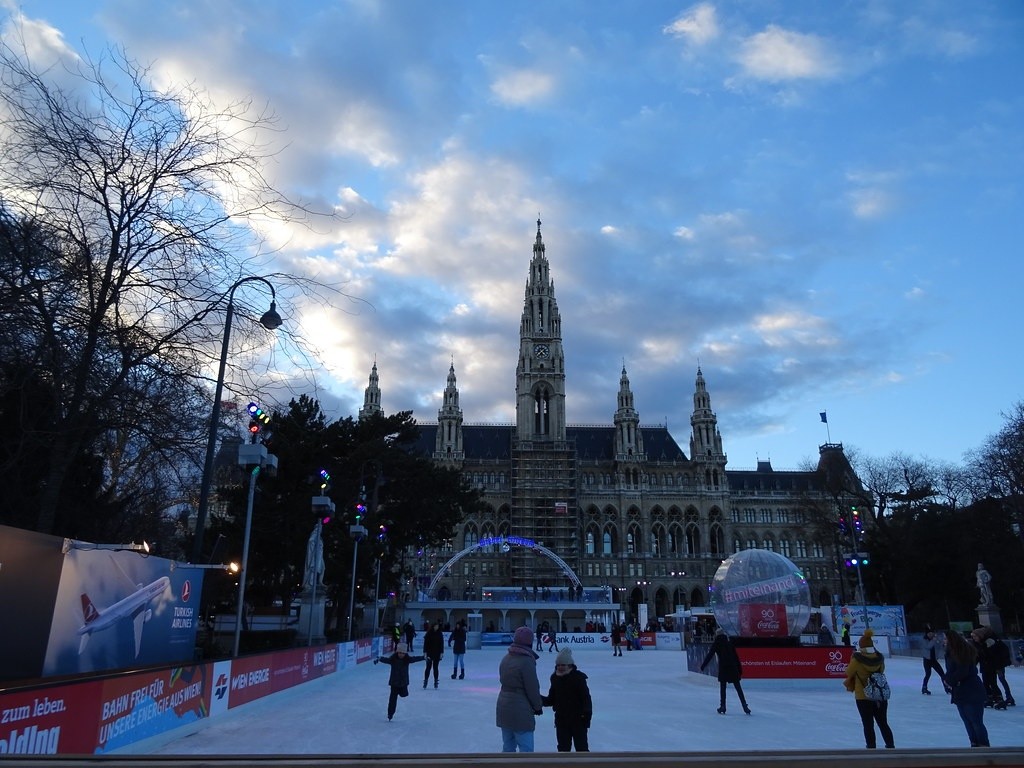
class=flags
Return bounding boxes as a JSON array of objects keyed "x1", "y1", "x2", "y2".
[{"x1": 820, "y1": 412, "x2": 826, "y2": 423}]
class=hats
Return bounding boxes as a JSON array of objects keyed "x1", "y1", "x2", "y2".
[
  {"x1": 396, "y1": 643, "x2": 408, "y2": 653},
  {"x1": 556, "y1": 647, "x2": 575, "y2": 665},
  {"x1": 514, "y1": 627, "x2": 533, "y2": 644},
  {"x1": 859, "y1": 629, "x2": 874, "y2": 648},
  {"x1": 972, "y1": 627, "x2": 985, "y2": 638}
]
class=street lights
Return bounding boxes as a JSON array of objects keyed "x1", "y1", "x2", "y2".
[
  {"x1": 600, "y1": 576, "x2": 610, "y2": 603},
  {"x1": 310, "y1": 498, "x2": 337, "y2": 648},
  {"x1": 843, "y1": 553, "x2": 870, "y2": 630},
  {"x1": 195, "y1": 275, "x2": 285, "y2": 565},
  {"x1": 232, "y1": 442, "x2": 280, "y2": 658},
  {"x1": 637, "y1": 583, "x2": 651, "y2": 603},
  {"x1": 670, "y1": 570, "x2": 686, "y2": 629},
  {"x1": 615, "y1": 587, "x2": 627, "y2": 610},
  {"x1": 467, "y1": 573, "x2": 475, "y2": 600},
  {"x1": 349, "y1": 526, "x2": 368, "y2": 642}
]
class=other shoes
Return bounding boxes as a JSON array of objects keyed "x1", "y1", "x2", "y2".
[
  {"x1": 984, "y1": 696, "x2": 1016, "y2": 710},
  {"x1": 424, "y1": 680, "x2": 428, "y2": 689},
  {"x1": 921, "y1": 687, "x2": 932, "y2": 695},
  {"x1": 434, "y1": 681, "x2": 438, "y2": 690},
  {"x1": 717, "y1": 706, "x2": 727, "y2": 714},
  {"x1": 744, "y1": 707, "x2": 751, "y2": 715},
  {"x1": 619, "y1": 654, "x2": 622, "y2": 656},
  {"x1": 945, "y1": 687, "x2": 952, "y2": 694},
  {"x1": 614, "y1": 653, "x2": 617, "y2": 656}
]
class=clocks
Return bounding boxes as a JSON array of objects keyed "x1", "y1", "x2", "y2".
[{"x1": 534, "y1": 344, "x2": 549, "y2": 359}]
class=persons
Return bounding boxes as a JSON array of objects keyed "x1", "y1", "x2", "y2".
[
  {"x1": 976, "y1": 563, "x2": 993, "y2": 606},
  {"x1": 685, "y1": 619, "x2": 715, "y2": 648},
  {"x1": 402, "y1": 618, "x2": 416, "y2": 652},
  {"x1": 842, "y1": 624, "x2": 850, "y2": 645},
  {"x1": 521, "y1": 583, "x2": 583, "y2": 602},
  {"x1": 644, "y1": 619, "x2": 684, "y2": 632},
  {"x1": 541, "y1": 648, "x2": 592, "y2": 752},
  {"x1": 394, "y1": 622, "x2": 403, "y2": 652},
  {"x1": 536, "y1": 624, "x2": 543, "y2": 651},
  {"x1": 490, "y1": 621, "x2": 494, "y2": 632},
  {"x1": 920, "y1": 623, "x2": 1015, "y2": 747},
  {"x1": 423, "y1": 618, "x2": 468, "y2": 690},
  {"x1": 610, "y1": 622, "x2": 642, "y2": 656},
  {"x1": 846, "y1": 628, "x2": 895, "y2": 749},
  {"x1": 310, "y1": 524, "x2": 326, "y2": 584},
  {"x1": 548, "y1": 626, "x2": 559, "y2": 652},
  {"x1": 574, "y1": 621, "x2": 606, "y2": 632},
  {"x1": 372, "y1": 643, "x2": 431, "y2": 722},
  {"x1": 496, "y1": 627, "x2": 542, "y2": 752},
  {"x1": 701, "y1": 628, "x2": 752, "y2": 714},
  {"x1": 563, "y1": 624, "x2": 567, "y2": 632},
  {"x1": 818, "y1": 623, "x2": 834, "y2": 645}
]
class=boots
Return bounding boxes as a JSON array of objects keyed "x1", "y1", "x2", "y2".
[
  {"x1": 459, "y1": 668, "x2": 464, "y2": 678},
  {"x1": 451, "y1": 668, "x2": 458, "y2": 678}
]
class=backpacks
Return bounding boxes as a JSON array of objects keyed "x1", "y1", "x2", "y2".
[{"x1": 856, "y1": 668, "x2": 890, "y2": 700}]
